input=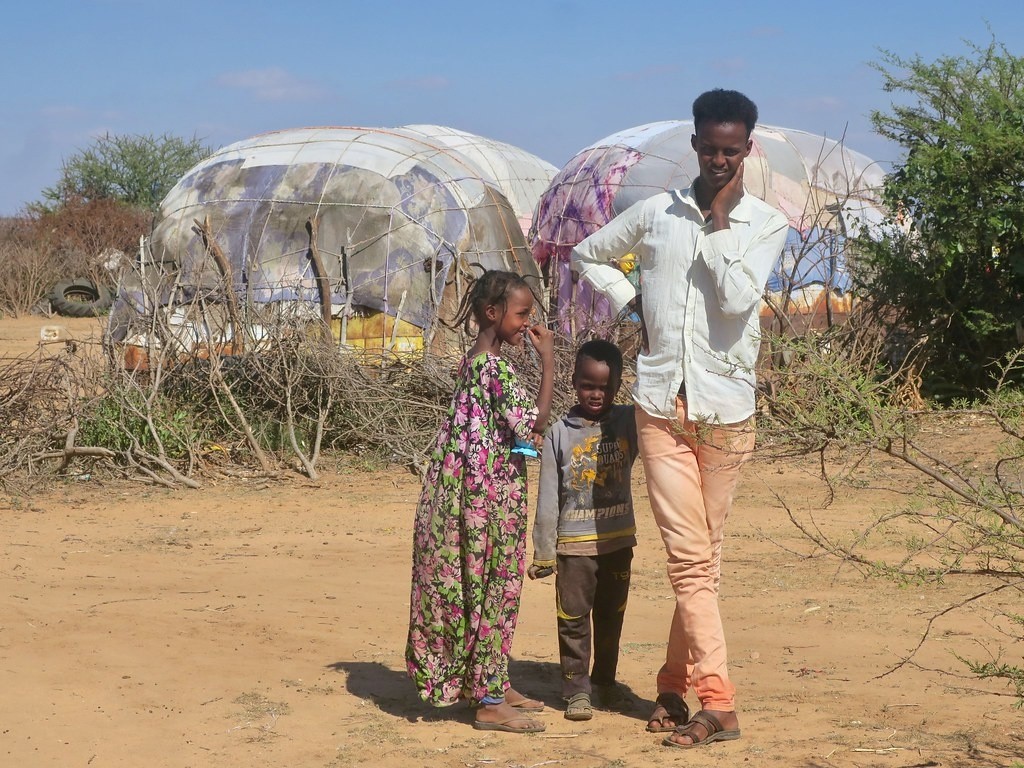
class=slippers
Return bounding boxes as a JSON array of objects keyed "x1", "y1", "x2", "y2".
[
  {"x1": 508, "y1": 698, "x2": 544, "y2": 711},
  {"x1": 475, "y1": 714, "x2": 546, "y2": 733}
]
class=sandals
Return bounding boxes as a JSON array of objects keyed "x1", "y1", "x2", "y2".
[
  {"x1": 645, "y1": 692, "x2": 689, "y2": 733},
  {"x1": 597, "y1": 686, "x2": 636, "y2": 711},
  {"x1": 565, "y1": 693, "x2": 592, "y2": 720},
  {"x1": 664, "y1": 710, "x2": 740, "y2": 749}
]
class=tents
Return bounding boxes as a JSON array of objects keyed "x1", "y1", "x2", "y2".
[
  {"x1": 103, "y1": 125, "x2": 561, "y2": 389},
  {"x1": 526, "y1": 121, "x2": 928, "y2": 317}
]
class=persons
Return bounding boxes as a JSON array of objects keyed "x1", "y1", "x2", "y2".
[
  {"x1": 525, "y1": 340, "x2": 641, "y2": 722},
  {"x1": 404, "y1": 261, "x2": 556, "y2": 732},
  {"x1": 568, "y1": 88, "x2": 790, "y2": 748}
]
